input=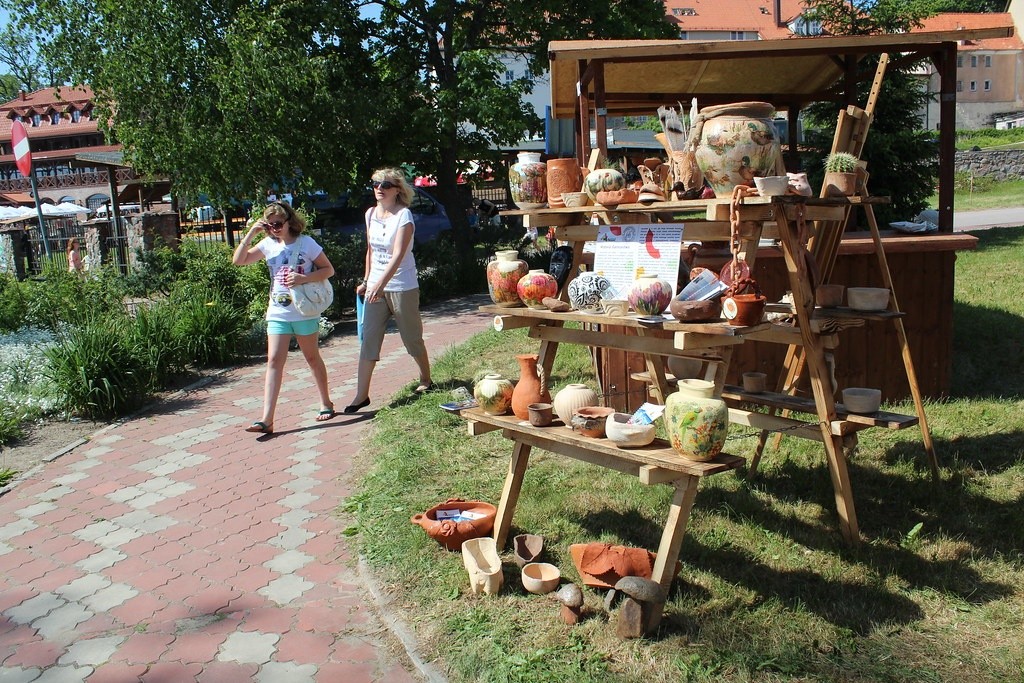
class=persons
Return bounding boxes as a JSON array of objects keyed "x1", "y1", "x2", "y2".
[
  {"x1": 233, "y1": 200, "x2": 335, "y2": 434},
  {"x1": 344, "y1": 169, "x2": 434, "y2": 414},
  {"x1": 465, "y1": 197, "x2": 501, "y2": 230},
  {"x1": 67, "y1": 237, "x2": 83, "y2": 278}
]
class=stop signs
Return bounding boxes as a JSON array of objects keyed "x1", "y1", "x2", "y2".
[{"x1": 11, "y1": 120, "x2": 31, "y2": 177}]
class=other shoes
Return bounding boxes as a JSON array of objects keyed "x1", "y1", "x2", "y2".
[
  {"x1": 414, "y1": 380, "x2": 435, "y2": 395},
  {"x1": 344, "y1": 397, "x2": 370, "y2": 413}
]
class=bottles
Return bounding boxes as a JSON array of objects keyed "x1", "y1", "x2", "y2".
[{"x1": 472, "y1": 99, "x2": 889, "y2": 464}]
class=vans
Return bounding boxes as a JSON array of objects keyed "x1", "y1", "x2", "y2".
[{"x1": 291, "y1": 186, "x2": 452, "y2": 255}]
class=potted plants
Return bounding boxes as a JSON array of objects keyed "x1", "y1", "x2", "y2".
[{"x1": 821, "y1": 151, "x2": 858, "y2": 196}]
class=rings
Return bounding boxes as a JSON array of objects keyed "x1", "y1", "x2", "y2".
[{"x1": 373, "y1": 292, "x2": 377, "y2": 296}]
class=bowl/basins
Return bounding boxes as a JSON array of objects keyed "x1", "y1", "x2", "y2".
[
  {"x1": 411, "y1": 498, "x2": 496, "y2": 549},
  {"x1": 521, "y1": 563, "x2": 561, "y2": 595}
]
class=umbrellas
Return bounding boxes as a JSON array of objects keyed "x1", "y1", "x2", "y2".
[
  {"x1": 0, "y1": 202, "x2": 92, "y2": 236},
  {"x1": 97, "y1": 196, "x2": 140, "y2": 216}
]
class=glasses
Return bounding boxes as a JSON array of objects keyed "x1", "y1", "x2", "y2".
[
  {"x1": 264, "y1": 215, "x2": 289, "y2": 230},
  {"x1": 371, "y1": 180, "x2": 400, "y2": 189}
]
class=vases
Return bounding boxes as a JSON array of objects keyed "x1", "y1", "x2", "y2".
[{"x1": 410, "y1": 99, "x2": 890, "y2": 591}]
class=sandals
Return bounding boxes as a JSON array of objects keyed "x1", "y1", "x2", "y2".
[
  {"x1": 244, "y1": 421, "x2": 273, "y2": 433},
  {"x1": 316, "y1": 402, "x2": 337, "y2": 421}
]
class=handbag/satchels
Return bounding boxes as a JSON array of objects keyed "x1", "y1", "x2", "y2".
[
  {"x1": 355, "y1": 292, "x2": 368, "y2": 342},
  {"x1": 289, "y1": 234, "x2": 334, "y2": 316}
]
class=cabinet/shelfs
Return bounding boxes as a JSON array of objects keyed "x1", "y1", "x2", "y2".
[{"x1": 460, "y1": 195, "x2": 939, "y2": 598}]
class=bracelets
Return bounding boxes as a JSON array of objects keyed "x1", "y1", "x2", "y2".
[
  {"x1": 241, "y1": 240, "x2": 251, "y2": 247},
  {"x1": 362, "y1": 280, "x2": 367, "y2": 287}
]
